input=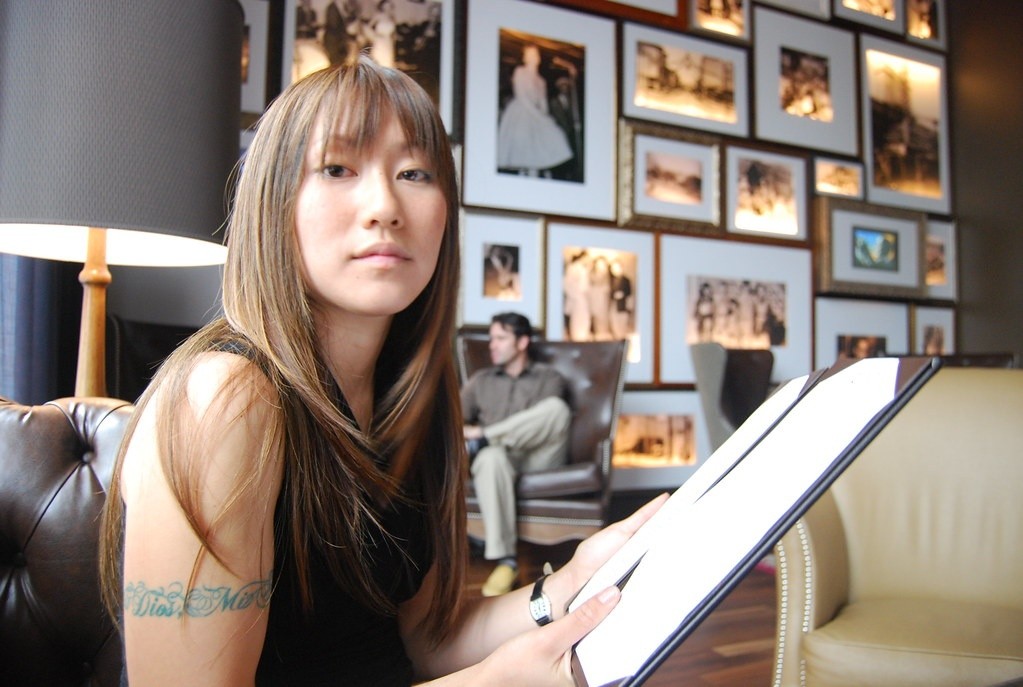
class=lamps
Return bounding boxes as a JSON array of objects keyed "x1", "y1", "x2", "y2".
[{"x1": 0, "y1": 1, "x2": 242, "y2": 400}]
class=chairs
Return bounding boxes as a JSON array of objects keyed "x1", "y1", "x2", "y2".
[
  {"x1": 456, "y1": 332, "x2": 630, "y2": 563},
  {"x1": 690, "y1": 343, "x2": 774, "y2": 454},
  {"x1": 108, "y1": 315, "x2": 203, "y2": 404}
]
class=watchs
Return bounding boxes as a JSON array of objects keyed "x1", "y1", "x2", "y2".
[{"x1": 529, "y1": 573, "x2": 554, "y2": 625}]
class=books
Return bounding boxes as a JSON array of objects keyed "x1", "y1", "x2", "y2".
[{"x1": 565, "y1": 352, "x2": 941, "y2": 687}]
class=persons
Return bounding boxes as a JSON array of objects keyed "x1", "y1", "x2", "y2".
[
  {"x1": 497, "y1": 42, "x2": 583, "y2": 180},
  {"x1": 455, "y1": 309, "x2": 573, "y2": 595},
  {"x1": 851, "y1": 338, "x2": 873, "y2": 358},
  {"x1": 96, "y1": 62, "x2": 671, "y2": 687},
  {"x1": 694, "y1": 280, "x2": 786, "y2": 344},
  {"x1": 296, "y1": 0, "x2": 441, "y2": 84},
  {"x1": 562, "y1": 249, "x2": 632, "y2": 343}
]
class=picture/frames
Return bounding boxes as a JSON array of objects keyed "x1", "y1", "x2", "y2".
[{"x1": 238, "y1": 0, "x2": 961, "y2": 392}]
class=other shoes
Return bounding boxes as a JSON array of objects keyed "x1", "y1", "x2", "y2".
[{"x1": 481, "y1": 564, "x2": 520, "y2": 597}]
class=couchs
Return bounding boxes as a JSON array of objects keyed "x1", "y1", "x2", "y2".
[
  {"x1": 0, "y1": 396, "x2": 140, "y2": 687},
  {"x1": 774, "y1": 367, "x2": 1023, "y2": 687}
]
